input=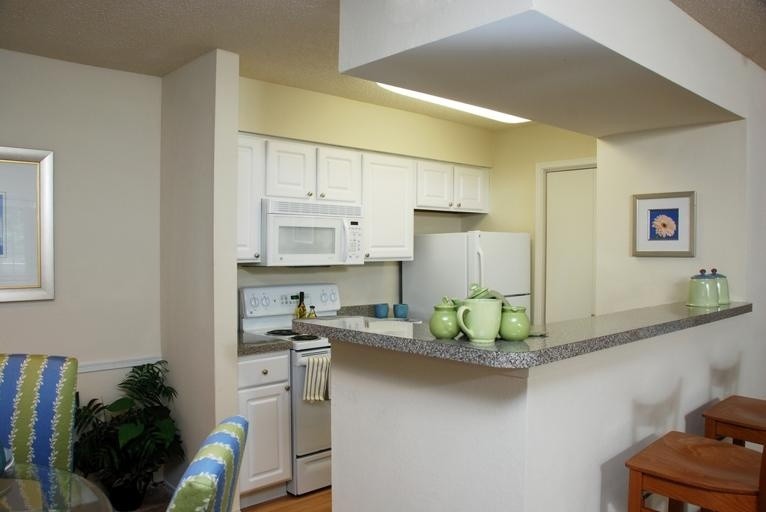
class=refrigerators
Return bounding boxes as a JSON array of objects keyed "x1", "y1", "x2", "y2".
[{"x1": 402, "y1": 231, "x2": 533, "y2": 338}]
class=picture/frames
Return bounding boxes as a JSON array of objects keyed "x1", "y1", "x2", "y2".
[
  {"x1": 0, "y1": 147, "x2": 55, "y2": 302},
  {"x1": 632, "y1": 190, "x2": 695, "y2": 258}
]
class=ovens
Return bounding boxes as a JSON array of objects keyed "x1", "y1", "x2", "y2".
[{"x1": 291, "y1": 349, "x2": 331, "y2": 495}]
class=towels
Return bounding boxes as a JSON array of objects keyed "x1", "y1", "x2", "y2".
[{"x1": 302, "y1": 355, "x2": 331, "y2": 404}]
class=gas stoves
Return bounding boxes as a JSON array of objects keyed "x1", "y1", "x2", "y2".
[{"x1": 254, "y1": 328, "x2": 330, "y2": 350}]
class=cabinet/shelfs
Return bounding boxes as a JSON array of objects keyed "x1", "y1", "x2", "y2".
[
  {"x1": 238, "y1": 132, "x2": 263, "y2": 262},
  {"x1": 415, "y1": 157, "x2": 490, "y2": 213},
  {"x1": 362, "y1": 151, "x2": 415, "y2": 262},
  {"x1": 266, "y1": 134, "x2": 362, "y2": 209},
  {"x1": 236, "y1": 326, "x2": 293, "y2": 510}
]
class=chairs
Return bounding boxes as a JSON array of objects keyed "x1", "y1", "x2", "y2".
[
  {"x1": 702, "y1": 394, "x2": 766, "y2": 455},
  {"x1": 167, "y1": 416, "x2": 246, "y2": 512},
  {"x1": 0, "y1": 353, "x2": 78, "y2": 473},
  {"x1": 624, "y1": 430, "x2": 765, "y2": 512}
]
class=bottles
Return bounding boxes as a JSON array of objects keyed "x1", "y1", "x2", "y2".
[
  {"x1": 308, "y1": 306, "x2": 318, "y2": 319},
  {"x1": 685, "y1": 268, "x2": 730, "y2": 308},
  {"x1": 500, "y1": 306, "x2": 531, "y2": 342},
  {"x1": 430, "y1": 296, "x2": 461, "y2": 340},
  {"x1": 296, "y1": 292, "x2": 307, "y2": 319}
]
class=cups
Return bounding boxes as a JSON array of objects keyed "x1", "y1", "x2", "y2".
[
  {"x1": 376, "y1": 303, "x2": 389, "y2": 318},
  {"x1": 393, "y1": 304, "x2": 409, "y2": 318},
  {"x1": 457, "y1": 298, "x2": 503, "y2": 343}
]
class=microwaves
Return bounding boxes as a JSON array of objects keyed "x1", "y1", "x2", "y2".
[{"x1": 242, "y1": 198, "x2": 365, "y2": 267}]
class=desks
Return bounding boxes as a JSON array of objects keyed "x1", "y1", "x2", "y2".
[{"x1": 0, "y1": 464, "x2": 112, "y2": 512}]
